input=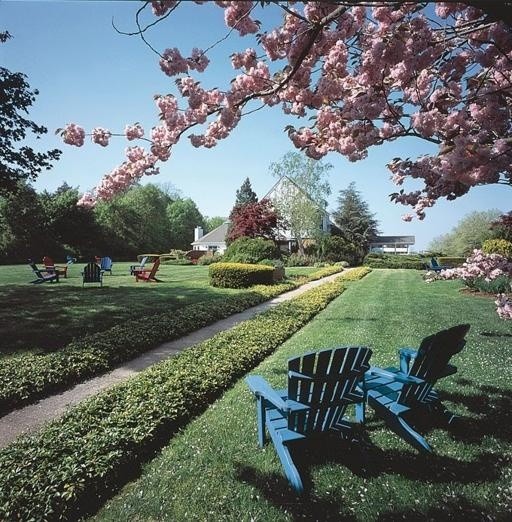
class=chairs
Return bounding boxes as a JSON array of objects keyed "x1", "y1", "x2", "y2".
[
  {"x1": 422, "y1": 261, "x2": 442, "y2": 273},
  {"x1": 30, "y1": 257, "x2": 160, "y2": 288},
  {"x1": 431, "y1": 259, "x2": 450, "y2": 272},
  {"x1": 245, "y1": 347, "x2": 384, "y2": 497},
  {"x1": 356, "y1": 324, "x2": 471, "y2": 454}
]
